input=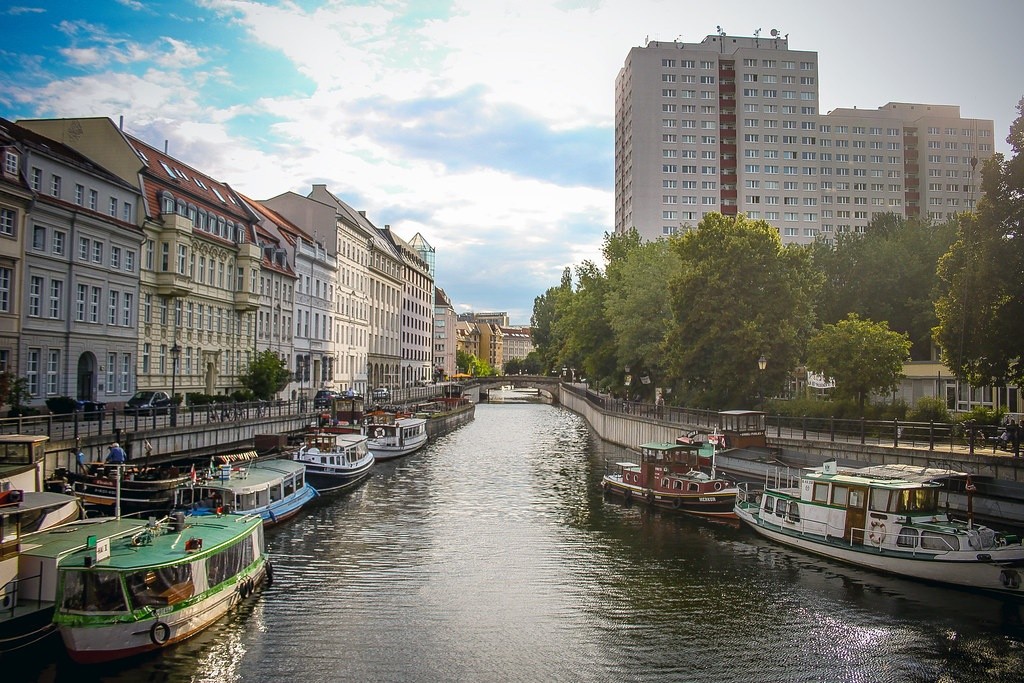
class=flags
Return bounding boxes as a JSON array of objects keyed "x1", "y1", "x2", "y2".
[{"x1": 190, "y1": 466, "x2": 196, "y2": 490}]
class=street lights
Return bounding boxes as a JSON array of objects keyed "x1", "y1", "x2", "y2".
[
  {"x1": 168, "y1": 341, "x2": 180, "y2": 426},
  {"x1": 299, "y1": 358, "x2": 307, "y2": 412}
]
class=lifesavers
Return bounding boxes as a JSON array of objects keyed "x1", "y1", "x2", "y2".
[
  {"x1": 248, "y1": 578, "x2": 255, "y2": 595},
  {"x1": 672, "y1": 497, "x2": 682, "y2": 509},
  {"x1": 623, "y1": 489, "x2": 632, "y2": 500},
  {"x1": 868, "y1": 520, "x2": 887, "y2": 544},
  {"x1": 240, "y1": 582, "x2": 250, "y2": 599},
  {"x1": 265, "y1": 561, "x2": 273, "y2": 578},
  {"x1": 646, "y1": 494, "x2": 654, "y2": 504},
  {"x1": 374, "y1": 427, "x2": 386, "y2": 439},
  {"x1": 713, "y1": 482, "x2": 722, "y2": 491},
  {"x1": 149, "y1": 620, "x2": 171, "y2": 646},
  {"x1": 603, "y1": 481, "x2": 610, "y2": 492}
]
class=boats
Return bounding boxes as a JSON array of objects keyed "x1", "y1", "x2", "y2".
[
  {"x1": 675, "y1": 410, "x2": 817, "y2": 491},
  {"x1": 366, "y1": 383, "x2": 475, "y2": 419},
  {"x1": 169, "y1": 459, "x2": 320, "y2": 526},
  {"x1": 0, "y1": 434, "x2": 53, "y2": 491},
  {"x1": 276, "y1": 396, "x2": 398, "y2": 450},
  {"x1": 52, "y1": 514, "x2": 275, "y2": 666},
  {"x1": 602, "y1": 441, "x2": 745, "y2": 520},
  {"x1": 46, "y1": 434, "x2": 306, "y2": 515},
  {"x1": 293, "y1": 433, "x2": 376, "y2": 492},
  {"x1": 366, "y1": 416, "x2": 429, "y2": 460},
  {"x1": 0, "y1": 491, "x2": 159, "y2": 661},
  {"x1": 733, "y1": 461, "x2": 1024, "y2": 601}
]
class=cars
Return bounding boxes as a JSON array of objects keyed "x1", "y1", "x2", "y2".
[
  {"x1": 312, "y1": 387, "x2": 393, "y2": 409},
  {"x1": 123, "y1": 390, "x2": 171, "y2": 416}
]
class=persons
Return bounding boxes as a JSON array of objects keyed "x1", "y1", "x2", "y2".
[
  {"x1": 655, "y1": 394, "x2": 664, "y2": 420},
  {"x1": 347, "y1": 387, "x2": 354, "y2": 399},
  {"x1": 102, "y1": 443, "x2": 126, "y2": 478},
  {"x1": 633, "y1": 393, "x2": 640, "y2": 402},
  {"x1": 996, "y1": 418, "x2": 1024, "y2": 453}
]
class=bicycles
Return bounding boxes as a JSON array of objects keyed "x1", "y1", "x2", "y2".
[{"x1": 203, "y1": 399, "x2": 271, "y2": 424}]
